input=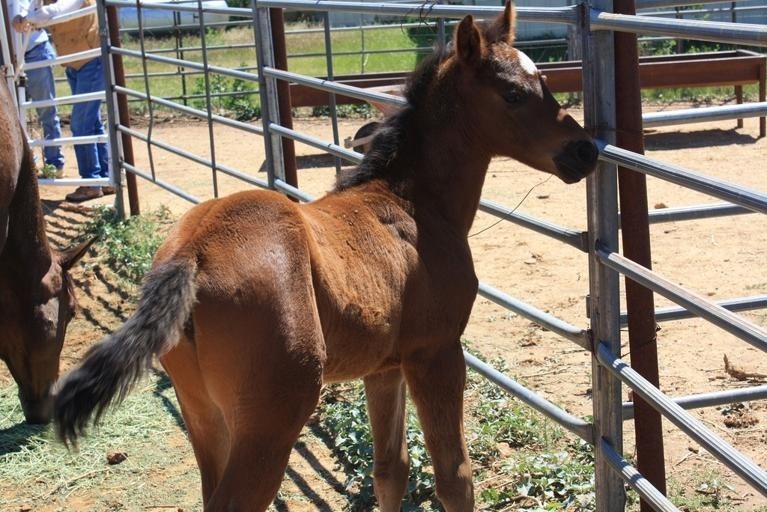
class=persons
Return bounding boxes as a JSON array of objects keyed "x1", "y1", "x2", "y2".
[
  {"x1": 0, "y1": 0, "x2": 64, "y2": 177},
  {"x1": 12, "y1": 0, "x2": 116, "y2": 203}
]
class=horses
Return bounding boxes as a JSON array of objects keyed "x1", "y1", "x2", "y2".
[
  {"x1": 47, "y1": 0, "x2": 601, "y2": 512},
  {"x1": 0, "y1": 63, "x2": 107, "y2": 426}
]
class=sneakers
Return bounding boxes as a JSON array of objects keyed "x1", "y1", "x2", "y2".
[{"x1": 66, "y1": 187, "x2": 112, "y2": 201}]
